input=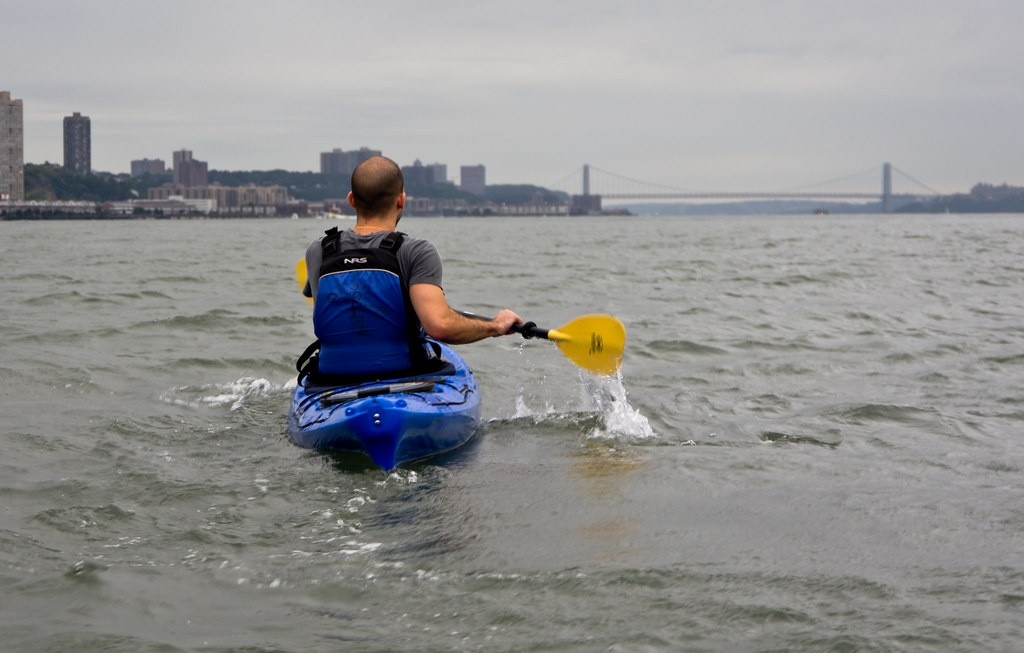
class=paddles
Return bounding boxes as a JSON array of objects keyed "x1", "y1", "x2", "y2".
[{"x1": 293, "y1": 258, "x2": 626, "y2": 377}]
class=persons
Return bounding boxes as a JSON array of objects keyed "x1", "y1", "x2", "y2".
[{"x1": 303, "y1": 156, "x2": 522, "y2": 385}]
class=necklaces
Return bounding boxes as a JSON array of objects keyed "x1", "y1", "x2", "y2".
[{"x1": 355, "y1": 226, "x2": 389, "y2": 231}]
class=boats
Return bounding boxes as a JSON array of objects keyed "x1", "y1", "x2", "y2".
[{"x1": 282, "y1": 331, "x2": 484, "y2": 470}]
